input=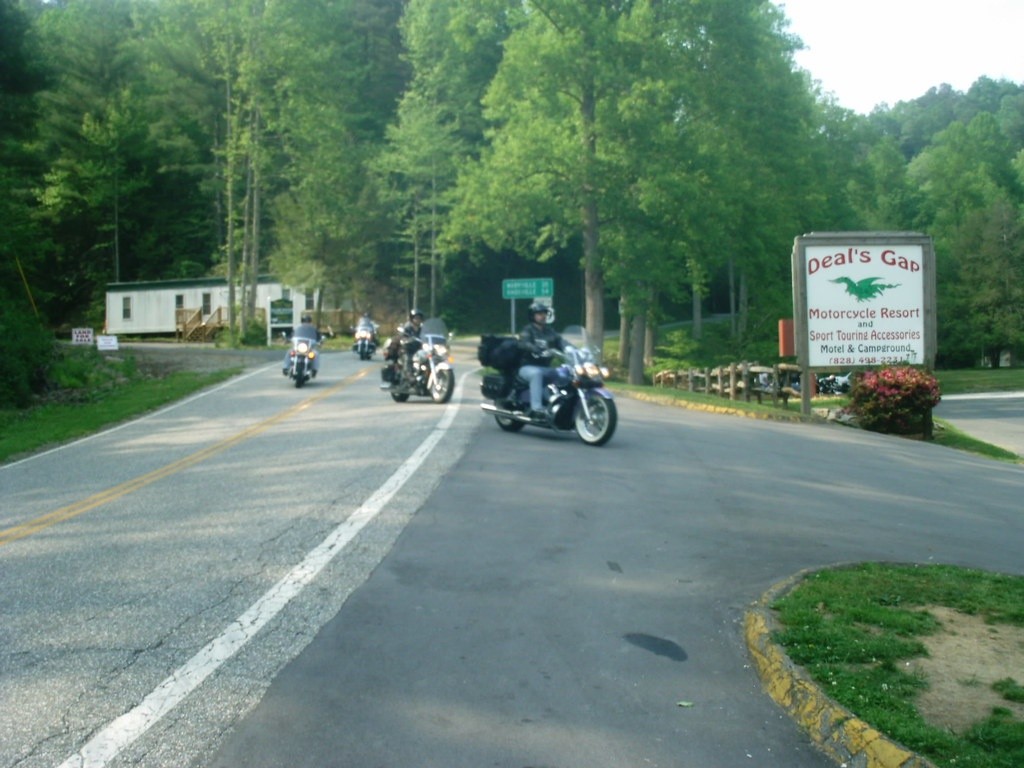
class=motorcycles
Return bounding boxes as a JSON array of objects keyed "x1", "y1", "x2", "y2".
[
  {"x1": 381, "y1": 315, "x2": 455, "y2": 404},
  {"x1": 480, "y1": 324, "x2": 618, "y2": 448},
  {"x1": 281, "y1": 324, "x2": 327, "y2": 388},
  {"x1": 350, "y1": 317, "x2": 379, "y2": 361}
]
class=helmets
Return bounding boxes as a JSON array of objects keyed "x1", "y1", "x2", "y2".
[
  {"x1": 302, "y1": 315, "x2": 311, "y2": 322},
  {"x1": 410, "y1": 310, "x2": 423, "y2": 321},
  {"x1": 364, "y1": 312, "x2": 370, "y2": 318},
  {"x1": 528, "y1": 303, "x2": 548, "y2": 321}
]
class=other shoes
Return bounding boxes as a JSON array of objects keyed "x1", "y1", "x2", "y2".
[{"x1": 531, "y1": 410, "x2": 547, "y2": 421}]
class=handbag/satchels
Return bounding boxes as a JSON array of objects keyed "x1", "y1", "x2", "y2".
[{"x1": 478, "y1": 333, "x2": 517, "y2": 370}]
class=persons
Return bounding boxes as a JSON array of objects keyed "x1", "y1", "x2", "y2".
[
  {"x1": 282, "y1": 315, "x2": 320, "y2": 377},
  {"x1": 518, "y1": 302, "x2": 577, "y2": 420},
  {"x1": 353, "y1": 311, "x2": 379, "y2": 352},
  {"x1": 398, "y1": 309, "x2": 424, "y2": 392}
]
